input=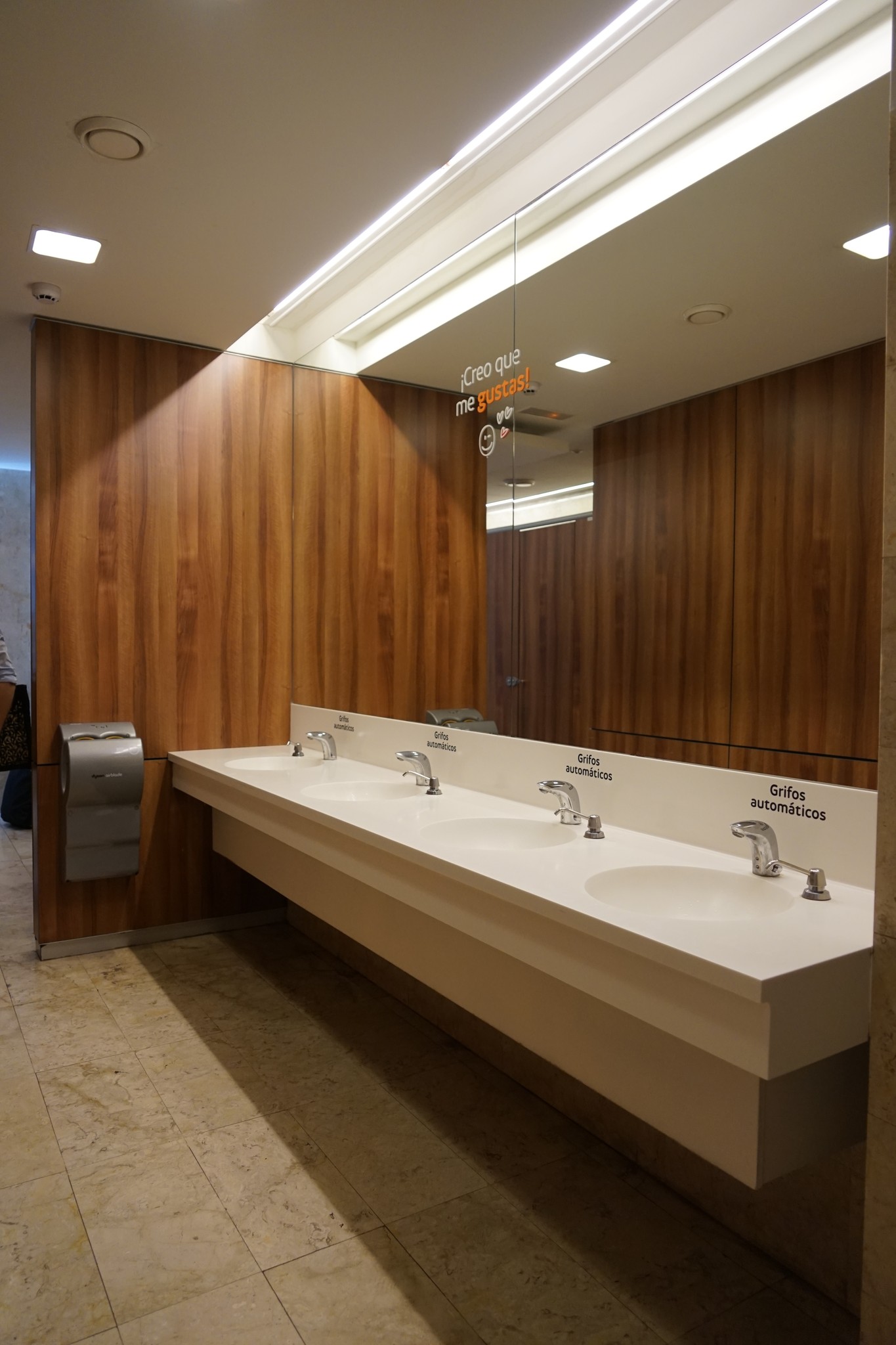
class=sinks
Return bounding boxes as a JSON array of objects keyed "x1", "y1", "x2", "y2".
[
  {"x1": 302, "y1": 781, "x2": 422, "y2": 803},
  {"x1": 225, "y1": 756, "x2": 325, "y2": 771},
  {"x1": 425, "y1": 817, "x2": 578, "y2": 849},
  {"x1": 587, "y1": 864, "x2": 798, "y2": 922}
]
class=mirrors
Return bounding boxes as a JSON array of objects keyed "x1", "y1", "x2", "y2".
[
  {"x1": 509, "y1": 55, "x2": 893, "y2": 800},
  {"x1": 289, "y1": 204, "x2": 519, "y2": 746}
]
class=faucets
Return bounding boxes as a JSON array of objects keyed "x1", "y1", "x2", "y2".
[
  {"x1": 287, "y1": 741, "x2": 304, "y2": 756},
  {"x1": 395, "y1": 751, "x2": 433, "y2": 787},
  {"x1": 538, "y1": 780, "x2": 581, "y2": 825},
  {"x1": 731, "y1": 819, "x2": 785, "y2": 877},
  {"x1": 554, "y1": 808, "x2": 606, "y2": 840},
  {"x1": 402, "y1": 771, "x2": 443, "y2": 796},
  {"x1": 306, "y1": 731, "x2": 337, "y2": 761},
  {"x1": 763, "y1": 859, "x2": 833, "y2": 901}
]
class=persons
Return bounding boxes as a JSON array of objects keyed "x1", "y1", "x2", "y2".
[{"x1": 0, "y1": 628, "x2": 18, "y2": 733}]
